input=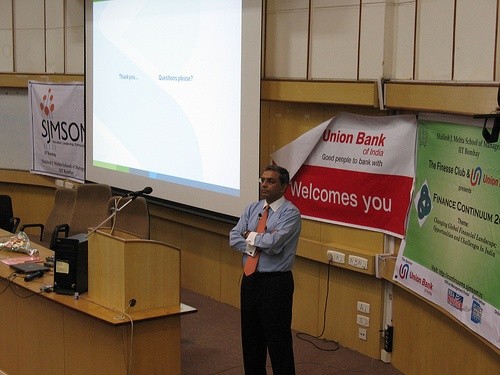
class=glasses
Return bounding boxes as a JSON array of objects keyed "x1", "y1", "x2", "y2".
[{"x1": 261, "y1": 178, "x2": 283, "y2": 185}]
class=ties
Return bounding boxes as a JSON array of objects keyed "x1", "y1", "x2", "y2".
[{"x1": 243, "y1": 205, "x2": 271, "y2": 277}]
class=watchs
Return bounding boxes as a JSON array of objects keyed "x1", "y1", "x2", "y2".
[{"x1": 242, "y1": 230, "x2": 252, "y2": 239}]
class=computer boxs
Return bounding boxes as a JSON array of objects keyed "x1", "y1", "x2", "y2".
[{"x1": 54, "y1": 232, "x2": 89, "y2": 294}]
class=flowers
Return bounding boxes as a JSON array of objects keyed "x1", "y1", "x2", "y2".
[{"x1": 0, "y1": 231, "x2": 39, "y2": 258}]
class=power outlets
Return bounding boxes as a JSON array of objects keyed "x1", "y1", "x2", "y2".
[
  {"x1": 348, "y1": 255, "x2": 368, "y2": 269},
  {"x1": 327, "y1": 249, "x2": 345, "y2": 264},
  {"x1": 357, "y1": 301, "x2": 370, "y2": 314},
  {"x1": 356, "y1": 314, "x2": 370, "y2": 327}
]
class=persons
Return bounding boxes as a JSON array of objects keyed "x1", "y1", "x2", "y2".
[{"x1": 229, "y1": 165, "x2": 302, "y2": 375}]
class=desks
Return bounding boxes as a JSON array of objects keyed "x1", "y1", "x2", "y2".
[{"x1": 0, "y1": 227, "x2": 198, "y2": 375}]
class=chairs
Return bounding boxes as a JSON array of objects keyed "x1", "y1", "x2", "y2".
[
  {"x1": 0, "y1": 194, "x2": 20, "y2": 232},
  {"x1": 105, "y1": 196, "x2": 150, "y2": 240},
  {"x1": 54, "y1": 182, "x2": 111, "y2": 247},
  {"x1": 19, "y1": 186, "x2": 78, "y2": 250}
]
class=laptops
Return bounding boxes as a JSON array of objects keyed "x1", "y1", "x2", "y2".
[{"x1": 10, "y1": 262, "x2": 50, "y2": 274}]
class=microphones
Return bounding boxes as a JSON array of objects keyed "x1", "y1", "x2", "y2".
[
  {"x1": 24, "y1": 270, "x2": 44, "y2": 282},
  {"x1": 122, "y1": 187, "x2": 153, "y2": 197}
]
